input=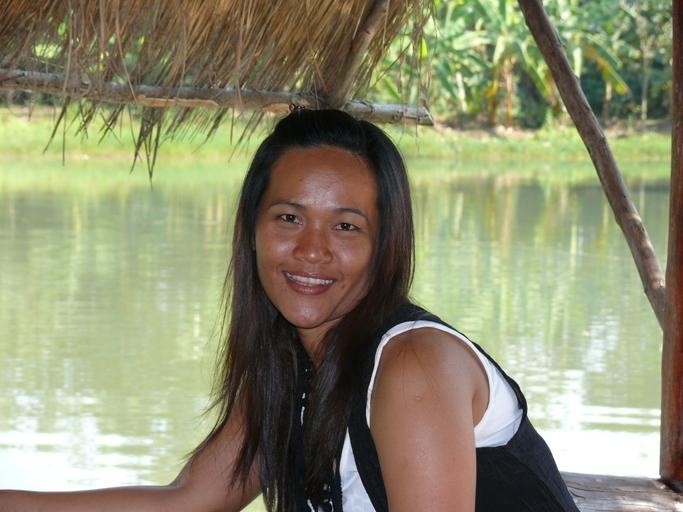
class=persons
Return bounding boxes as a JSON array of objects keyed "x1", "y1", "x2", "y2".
[{"x1": 1, "y1": 103, "x2": 580, "y2": 512}]
